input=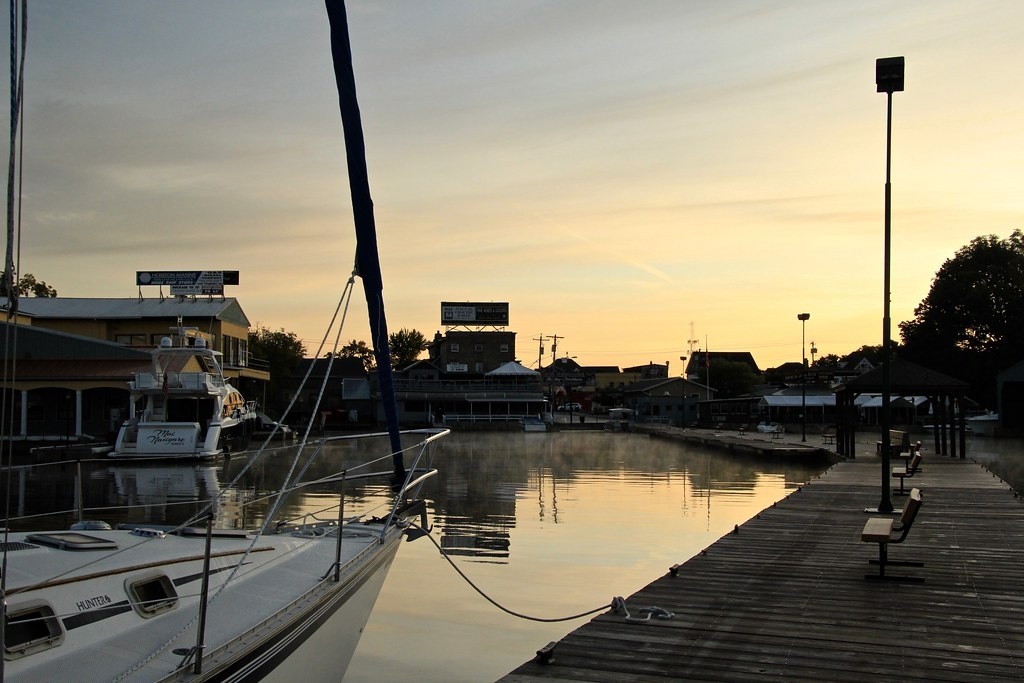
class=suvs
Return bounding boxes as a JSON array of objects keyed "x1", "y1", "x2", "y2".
[{"x1": 558, "y1": 403, "x2": 582, "y2": 412}]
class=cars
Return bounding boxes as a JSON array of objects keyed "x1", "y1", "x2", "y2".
[
  {"x1": 690, "y1": 420, "x2": 703, "y2": 428},
  {"x1": 757, "y1": 422, "x2": 785, "y2": 433},
  {"x1": 716, "y1": 423, "x2": 730, "y2": 429},
  {"x1": 738, "y1": 424, "x2": 753, "y2": 431}
]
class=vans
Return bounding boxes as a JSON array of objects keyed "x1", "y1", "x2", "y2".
[{"x1": 609, "y1": 408, "x2": 633, "y2": 418}]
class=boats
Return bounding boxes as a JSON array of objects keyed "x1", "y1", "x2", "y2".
[
  {"x1": 923, "y1": 409, "x2": 998, "y2": 437},
  {"x1": 107, "y1": 314, "x2": 258, "y2": 461},
  {"x1": 518, "y1": 419, "x2": 546, "y2": 433}
]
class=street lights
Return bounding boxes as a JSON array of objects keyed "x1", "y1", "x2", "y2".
[
  {"x1": 798, "y1": 313, "x2": 810, "y2": 442},
  {"x1": 864, "y1": 57, "x2": 904, "y2": 513},
  {"x1": 680, "y1": 356, "x2": 687, "y2": 430}
]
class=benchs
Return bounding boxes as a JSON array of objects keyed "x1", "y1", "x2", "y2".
[
  {"x1": 875, "y1": 429, "x2": 911, "y2": 460},
  {"x1": 737, "y1": 424, "x2": 749, "y2": 436},
  {"x1": 859, "y1": 487, "x2": 930, "y2": 582},
  {"x1": 899, "y1": 440, "x2": 923, "y2": 473},
  {"x1": 768, "y1": 425, "x2": 785, "y2": 440},
  {"x1": 665, "y1": 420, "x2": 678, "y2": 430},
  {"x1": 690, "y1": 421, "x2": 699, "y2": 431},
  {"x1": 891, "y1": 451, "x2": 924, "y2": 497},
  {"x1": 713, "y1": 423, "x2": 725, "y2": 433},
  {"x1": 821, "y1": 428, "x2": 837, "y2": 445}
]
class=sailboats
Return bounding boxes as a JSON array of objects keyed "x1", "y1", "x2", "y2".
[{"x1": 0, "y1": 0, "x2": 451, "y2": 683}]
